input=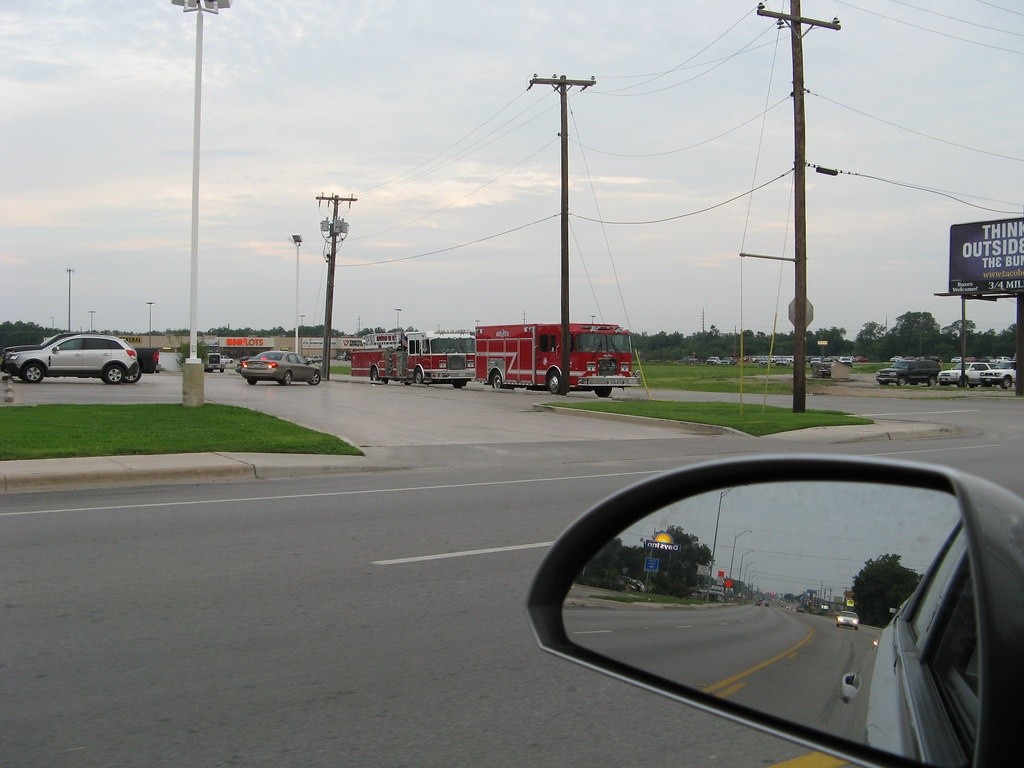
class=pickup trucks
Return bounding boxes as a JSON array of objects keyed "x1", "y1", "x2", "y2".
[{"x1": 2, "y1": 333, "x2": 161, "y2": 383}]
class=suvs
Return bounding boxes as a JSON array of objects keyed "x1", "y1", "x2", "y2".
[
  {"x1": 876, "y1": 359, "x2": 942, "y2": 387},
  {"x1": 221, "y1": 355, "x2": 233, "y2": 364},
  {"x1": 937, "y1": 362, "x2": 1000, "y2": 387},
  {"x1": 4, "y1": 334, "x2": 138, "y2": 384},
  {"x1": 979, "y1": 362, "x2": 1017, "y2": 390}
]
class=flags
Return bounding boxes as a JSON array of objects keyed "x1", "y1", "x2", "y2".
[{"x1": 402, "y1": 336, "x2": 408, "y2": 347}]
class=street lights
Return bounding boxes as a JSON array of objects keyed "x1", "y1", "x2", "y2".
[
  {"x1": 170, "y1": 0, "x2": 236, "y2": 406},
  {"x1": 291, "y1": 234, "x2": 302, "y2": 355},
  {"x1": 89, "y1": 310, "x2": 96, "y2": 332},
  {"x1": 396, "y1": 308, "x2": 401, "y2": 328},
  {"x1": 589, "y1": 315, "x2": 596, "y2": 324},
  {"x1": 726, "y1": 529, "x2": 757, "y2": 602},
  {"x1": 146, "y1": 301, "x2": 154, "y2": 347}
]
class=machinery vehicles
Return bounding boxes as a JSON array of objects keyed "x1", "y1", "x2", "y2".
[{"x1": 199, "y1": 335, "x2": 226, "y2": 373}]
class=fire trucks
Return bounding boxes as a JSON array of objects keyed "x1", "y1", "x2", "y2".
[
  {"x1": 475, "y1": 324, "x2": 642, "y2": 397},
  {"x1": 351, "y1": 332, "x2": 476, "y2": 388}
]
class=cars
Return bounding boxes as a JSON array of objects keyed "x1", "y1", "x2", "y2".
[
  {"x1": 837, "y1": 610, "x2": 859, "y2": 630},
  {"x1": 797, "y1": 606, "x2": 804, "y2": 612},
  {"x1": 890, "y1": 356, "x2": 1017, "y2": 362},
  {"x1": 828, "y1": 517, "x2": 979, "y2": 766},
  {"x1": 337, "y1": 354, "x2": 348, "y2": 361},
  {"x1": 235, "y1": 351, "x2": 322, "y2": 385},
  {"x1": 679, "y1": 355, "x2": 868, "y2": 378}
]
